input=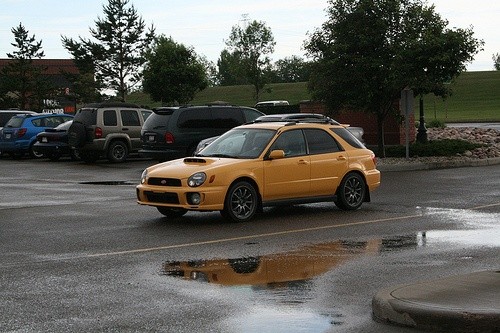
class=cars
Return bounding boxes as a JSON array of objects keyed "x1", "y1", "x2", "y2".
[
  {"x1": 195, "y1": 114, "x2": 365, "y2": 154},
  {"x1": 0, "y1": 114, "x2": 75, "y2": 158},
  {"x1": 138, "y1": 122, "x2": 382, "y2": 223},
  {"x1": 34, "y1": 120, "x2": 79, "y2": 162}
]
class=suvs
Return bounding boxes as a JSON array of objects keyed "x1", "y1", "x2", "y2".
[
  {"x1": 140, "y1": 104, "x2": 267, "y2": 160},
  {"x1": 67, "y1": 104, "x2": 157, "y2": 163}
]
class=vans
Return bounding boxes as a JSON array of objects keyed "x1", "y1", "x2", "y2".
[{"x1": 253, "y1": 100, "x2": 290, "y2": 107}]
